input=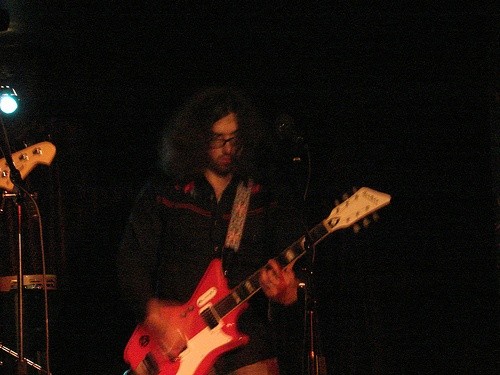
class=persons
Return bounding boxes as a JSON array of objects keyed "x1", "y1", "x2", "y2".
[{"x1": 114, "y1": 86, "x2": 297, "y2": 375}]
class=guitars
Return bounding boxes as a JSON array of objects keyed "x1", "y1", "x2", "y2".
[
  {"x1": 123, "y1": 187, "x2": 391, "y2": 375},
  {"x1": 1, "y1": 136, "x2": 56, "y2": 197}
]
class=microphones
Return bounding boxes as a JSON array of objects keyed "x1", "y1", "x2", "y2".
[{"x1": 273, "y1": 114, "x2": 305, "y2": 148}]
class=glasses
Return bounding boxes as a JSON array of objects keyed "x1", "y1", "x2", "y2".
[{"x1": 205, "y1": 135, "x2": 243, "y2": 150}]
